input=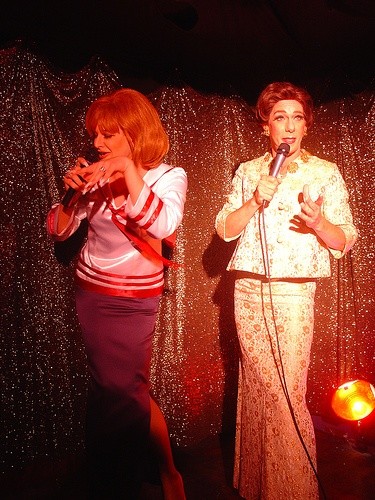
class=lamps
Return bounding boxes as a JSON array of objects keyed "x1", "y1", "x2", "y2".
[{"x1": 329, "y1": 377, "x2": 375, "y2": 449}]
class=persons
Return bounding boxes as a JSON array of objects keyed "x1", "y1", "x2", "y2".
[
  {"x1": 46, "y1": 88, "x2": 188, "y2": 500},
  {"x1": 215, "y1": 83, "x2": 359, "y2": 500}
]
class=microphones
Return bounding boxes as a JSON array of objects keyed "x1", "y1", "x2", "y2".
[
  {"x1": 61, "y1": 150, "x2": 98, "y2": 207},
  {"x1": 262, "y1": 143, "x2": 290, "y2": 208}
]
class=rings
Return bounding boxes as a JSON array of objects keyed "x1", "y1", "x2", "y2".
[{"x1": 99, "y1": 167, "x2": 106, "y2": 172}]
